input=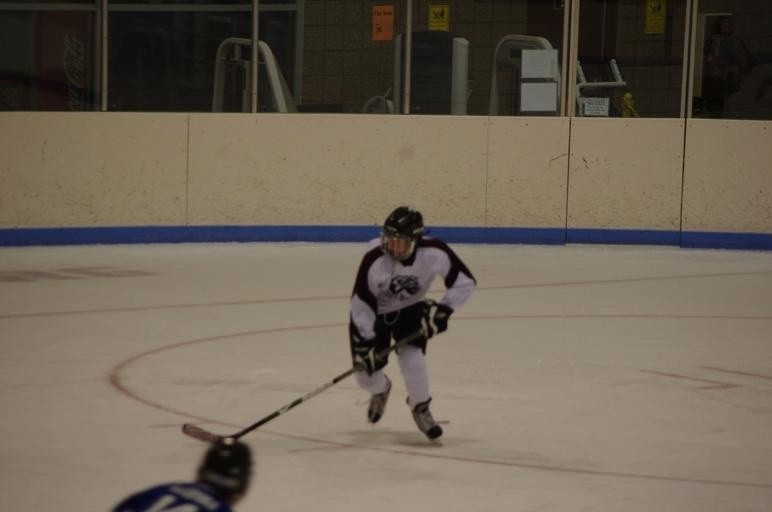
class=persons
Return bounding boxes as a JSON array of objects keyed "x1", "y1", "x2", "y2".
[
  {"x1": 348, "y1": 205, "x2": 478, "y2": 440},
  {"x1": 110, "y1": 436, "x2": 253, "y2": 512}
]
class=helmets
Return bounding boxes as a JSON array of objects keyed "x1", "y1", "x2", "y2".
[
  {"x1": 379, "y1": 205, "x2": 424, "y2": 260},
  {"x1": 194, "y1": 436, "x2": 253, "y2": 496}
]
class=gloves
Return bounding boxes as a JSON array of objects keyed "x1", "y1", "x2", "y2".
[
  {"x1": 417, "y1": 301, "x2": 452, "y2": 338},
  {"x1": 347, "y1": 334, "x2": 388, "y2": 376}
]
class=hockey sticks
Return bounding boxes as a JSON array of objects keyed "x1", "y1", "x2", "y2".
[{"x1": 182, "y1": 329, "x2": 422, "y2": 442}]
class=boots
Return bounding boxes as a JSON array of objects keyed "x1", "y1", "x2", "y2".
[
  {"x1": 366, "y1": 374, "x2": 392, "y2": 424},
  {"x1": 405, "y1": 395, "x2": 442, "y2": 439}
]
